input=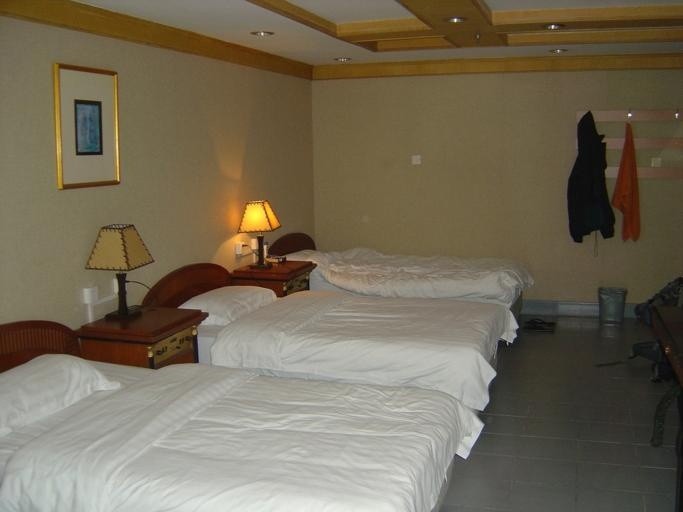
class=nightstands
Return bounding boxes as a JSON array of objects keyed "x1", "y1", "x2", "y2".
[
  {"x1": 75, "y1": 304, "x2": 209, "y2": 370},
  {"x1": 231, "y1": 261, "x2": 317, "y2": 298}
]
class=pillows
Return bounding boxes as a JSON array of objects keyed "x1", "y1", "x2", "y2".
[
  {"x1": 282, "y1": 249, "x2": 315, "y2": 261},
  {"x1": 0, "y1": 353, "x2": 121, "y2": 438},
  {"x1": 176, "y1": 286, "x2": 278, "y2": 327}
]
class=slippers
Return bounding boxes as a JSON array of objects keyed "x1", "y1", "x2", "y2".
[{"x1": 525, "y1": 318, "x2": 554, "y2": 332}]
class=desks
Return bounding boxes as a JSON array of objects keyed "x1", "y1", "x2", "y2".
[{"x1": 651, "y1": 304, "x2": 683, "y2": 447}]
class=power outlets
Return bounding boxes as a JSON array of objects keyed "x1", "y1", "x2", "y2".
[
  {"x1": 235, "y1": 242, "x2": 246, "y2": 254},
  {"x1": 113, "y1": 278, "x2": 128, "y2": 295}
]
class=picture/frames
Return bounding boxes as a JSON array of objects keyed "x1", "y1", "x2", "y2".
[{"x1": 53, "y1": 62, "x2": 121, "y2": 190}]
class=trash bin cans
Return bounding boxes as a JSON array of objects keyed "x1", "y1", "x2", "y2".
[{"x1": 598, "y1": 287, "x2": 627, "y2": 324}]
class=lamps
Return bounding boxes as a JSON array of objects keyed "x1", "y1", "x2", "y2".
[
  {"x1": 238, "y1": 200, "x2": 281, "y2": 270},
  {"x1": 85, "y1": 224, "x2": 155, "y2": 321}
]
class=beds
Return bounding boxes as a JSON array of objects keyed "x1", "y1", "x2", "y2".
[
  {"x1": 0, "y1": 320, "x2": 485, "y2": 512},
  {"x1": 140, "y1": 263, "x2": 519, "y2": 417},
  {"x1": 268, "y1": 233, "x2": 535, "y2": 321}
]
